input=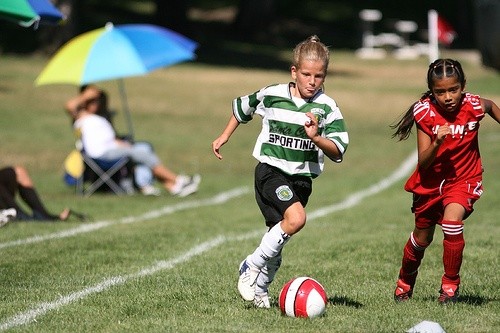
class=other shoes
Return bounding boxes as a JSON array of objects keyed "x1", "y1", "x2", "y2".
[
  {"x1": 171, "y1": 174, "x2": 202, "y2": 198},
  {"x1": 142, "y1": 185, "x2": 161, "y2": 197},
  {"x1": 437, "y1": 283, "x2": 460, "y2": 305},
  {"x1": 393, "y1": 277, "x2": 416, "y2": 305}
]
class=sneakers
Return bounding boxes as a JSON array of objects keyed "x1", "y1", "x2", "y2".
[
  {"x1": 237, "y1": 256, "x2": 261, "y2": 302},
  {"x1": 253, "y1": 293, "x2": 271, "y2": 310}
]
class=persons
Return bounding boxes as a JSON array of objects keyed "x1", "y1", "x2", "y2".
[
  {"x1": 389, "y1": 58, "x2": 500, "y2": 304},
  {"x1": 211, "y1": 36, "x2": 348, "y2": 308},
  {"x1": 0, "y1": 166, "x2": 70, "y2": 220},
  {"x1": 64, "y1": 83, "x2": 200, "y2": 197}
]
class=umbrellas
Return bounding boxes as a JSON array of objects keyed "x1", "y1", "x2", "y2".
[
  {"x1": 34, "y1": 22, "x2": 197, "y2": 145},
  {"x1": 0, "y1": 0, "x2": 62, "y2": 26}
]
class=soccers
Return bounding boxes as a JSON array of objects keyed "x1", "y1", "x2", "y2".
[{"x1": 278, "y1": 276, "x2": 328, "y2": 319}]
